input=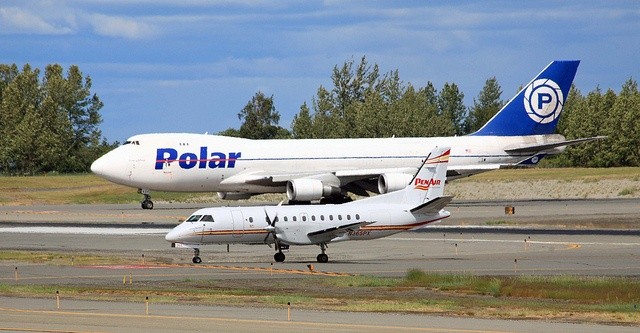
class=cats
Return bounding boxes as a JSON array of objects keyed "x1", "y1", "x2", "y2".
[
  {"x1": 91, "y1": 59, "x2": 608, "y2": 209},
  {"x1": 164, "y1": 144, "x2": 453, "y2": 264}
]
